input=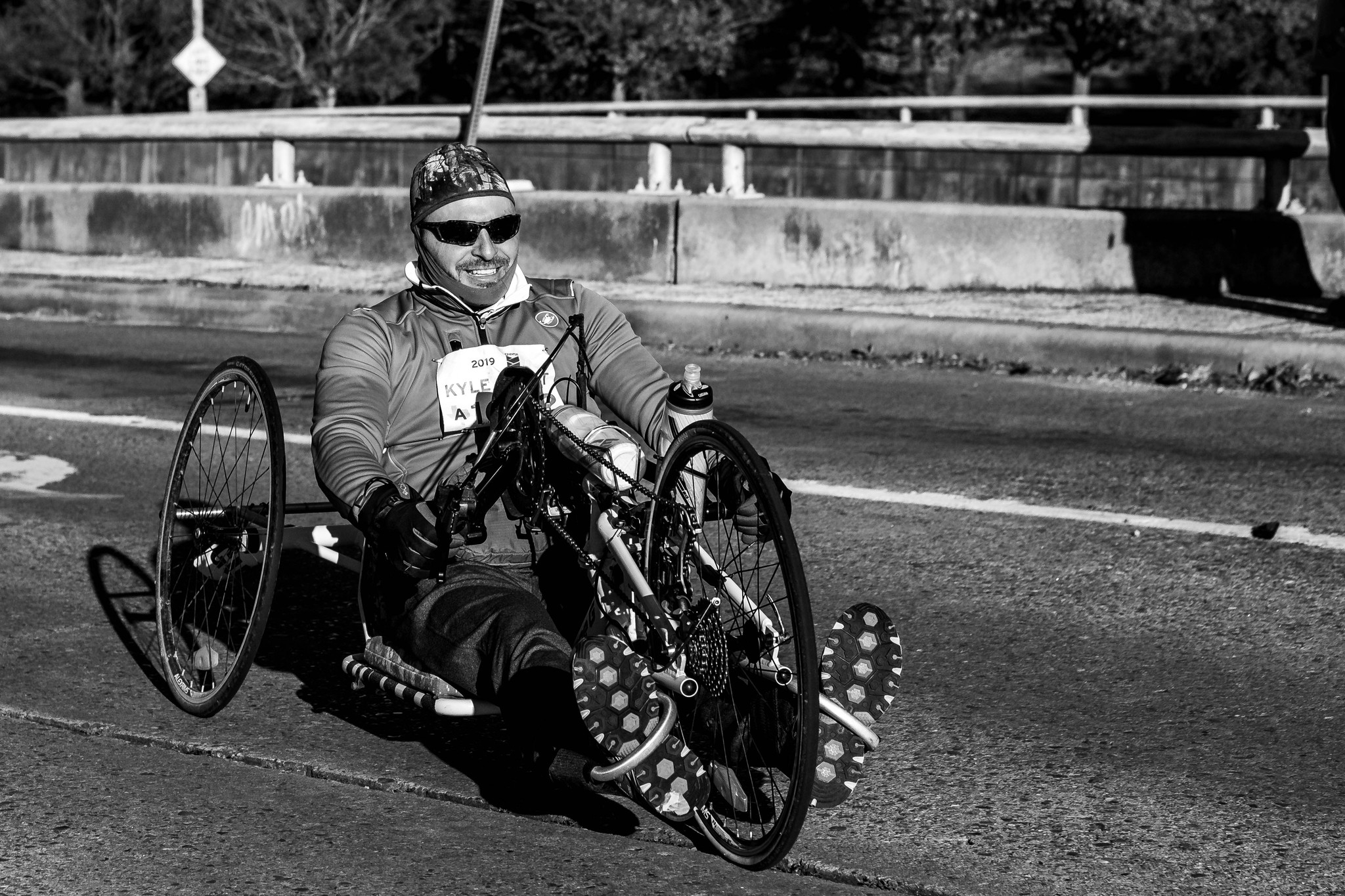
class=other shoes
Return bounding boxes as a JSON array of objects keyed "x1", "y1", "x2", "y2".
[
  {"x1": 571, "y1": 634, "x2": 713, "y2": 824},
  {"x1": 809, "y1": 603, "x2": 902, "y2": 809}
]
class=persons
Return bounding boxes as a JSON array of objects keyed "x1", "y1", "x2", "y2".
[{"x1": 311, "y1": 143, "x2": 902, "y2": 822}]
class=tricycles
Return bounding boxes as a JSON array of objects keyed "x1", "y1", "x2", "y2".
[{"x1": 151, "y1": 311, "x2": 884, "y2": 878}]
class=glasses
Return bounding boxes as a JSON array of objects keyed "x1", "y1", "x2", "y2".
[{"x1": 417, "y1": 212, "x2": 521, "y2": 246}]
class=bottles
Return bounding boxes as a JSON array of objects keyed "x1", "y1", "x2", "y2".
[
  {"x1": 542, "y1": 404, "x2": 645, "y2": 491},
  {"x1": 657, "y1": 362, "x2": 715, "y2": 533}
]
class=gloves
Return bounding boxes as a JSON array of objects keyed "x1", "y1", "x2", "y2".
[
  {"x1": 707, "y1": 455, "x2": 793, "y2": 545},
  {"x1": 355, "y1": 479, "x2": 465, "y2": 582}
]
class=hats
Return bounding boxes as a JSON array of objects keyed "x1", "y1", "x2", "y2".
[{"x1": 410, "y1": 142, "x2": 514, "y2": 231}]
